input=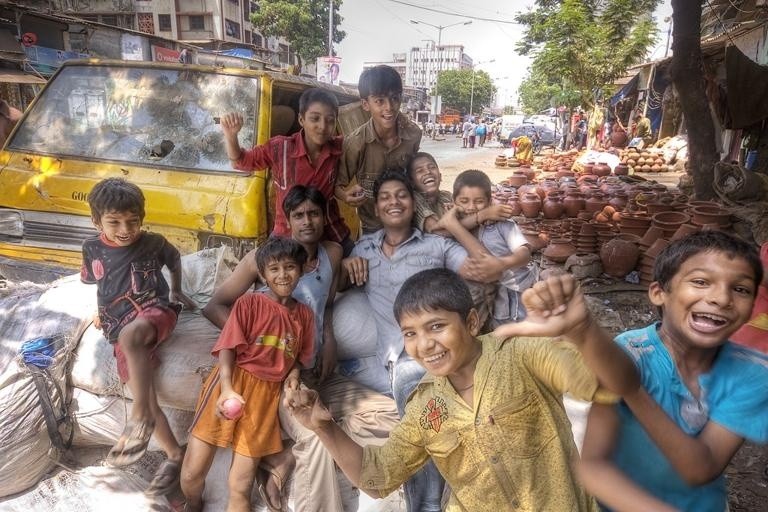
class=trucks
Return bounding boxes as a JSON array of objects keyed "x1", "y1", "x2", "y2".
[{"x1": 497, "y1": 113, "x2": 563, "y2": 149}]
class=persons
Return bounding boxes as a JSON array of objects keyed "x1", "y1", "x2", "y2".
[
  {"x1": 448, "y1": 168, "x2": 544, "y2": 335},
  {"x1": 221, "y1": 85, "x2": 356, "y2": 256},
  {"x1": 279, "y1": 264, "x2": 642, "y2": 512},
  {"x1": 176, "y1": 235, "x2": 317, "y2": 512},
  {"x1": 328, "y1": 64, "x2": 340, "y2": 85},
  {"x1": 569, "y1": 226, "x2": 768, "y2": 511},
  {"x1": 333, "y1": 174, "x2": 503, "y2": 423},
  {"x1": 331, "y1": 62, "x2": 425, "y2": 234},
  {"x1": 414, "y1": 107, "x2": 606, "y2": 150},
  {"x1": 508, "y1": 135, "x2": 536, "y2": 164},
  {"x1": 80, "y1": 177, "x2": 199, "y2": 497},
  {"x1": 407, "y1": 151, "x2": 516, "y2": 237},
  {"x1": 200, "y1": 180, "x2": 346, "y2": 389},
  {"x1": 633, "y1": 114, "x2": 653, "y2": 147}
]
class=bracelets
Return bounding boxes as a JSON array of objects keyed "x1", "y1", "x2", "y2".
[{"x1": 474, "y1": 212, "x2": 483, "y2": 228}]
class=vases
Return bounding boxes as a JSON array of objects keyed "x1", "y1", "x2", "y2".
[{"x1": 543, "y1": 202, "x2": 732, "y2": 289}]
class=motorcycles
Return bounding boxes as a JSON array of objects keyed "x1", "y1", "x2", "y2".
[{"x1": 530, "y1": 126, "x2": 546, "y2": 157}]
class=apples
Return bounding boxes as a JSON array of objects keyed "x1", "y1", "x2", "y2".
[{"x1": 223, "y1": 398, "x2": 243, "y2": 419}]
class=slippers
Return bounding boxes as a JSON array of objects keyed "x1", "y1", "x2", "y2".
[
  {"x1": 144, "y1": 444, "x2": 188, "y2": 497},
  {"x1": 255, "y1": 461, "x2": 286, "y2": 512},
  {"x1": 107, "y1": 419, "x2": 155, "y2": 467}
]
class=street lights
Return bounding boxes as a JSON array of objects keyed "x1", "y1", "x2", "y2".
[
  {"x1": 410, "y1": 18, "x2": 474, "y2": 140},
  {"x1": 469, "y1": 58, "x2": 515, "y2": 126}
]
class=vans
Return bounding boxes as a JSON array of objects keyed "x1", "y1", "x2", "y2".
[{"x1": 3, "y1": 55, "x2": 373, "y2": 303}]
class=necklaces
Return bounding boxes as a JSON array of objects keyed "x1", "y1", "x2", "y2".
[{"x1": 452, "y1": 378, "x2": 479, "y2": 397}]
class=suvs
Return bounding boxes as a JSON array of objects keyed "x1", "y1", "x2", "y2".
[{"x1": 507, "y1": 126, "x2": 559, "y2": 150}]
